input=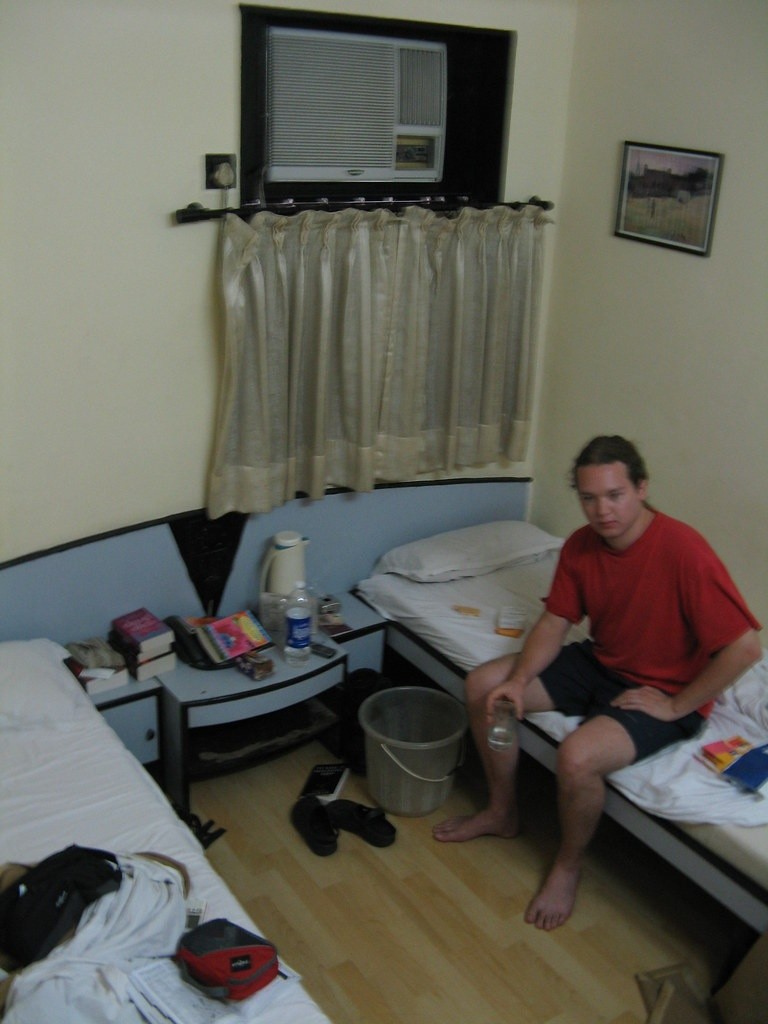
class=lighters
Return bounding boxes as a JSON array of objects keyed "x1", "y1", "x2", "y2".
[{"x1": 451, "y1": 605, "x2": 481, "y2": 617}]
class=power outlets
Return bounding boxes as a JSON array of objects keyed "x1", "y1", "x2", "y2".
[{"x1": 204, "y1": 154, "x2": 237, "y2": 188}]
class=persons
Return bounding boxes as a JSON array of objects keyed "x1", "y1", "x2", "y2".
[{"x1": 432, "y1": 436, "x2": 762, "y2": 930}]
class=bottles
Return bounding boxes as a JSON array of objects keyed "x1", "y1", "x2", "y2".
[{"x1": 283, "y1": 580, "x2": 312, "y2": 668}]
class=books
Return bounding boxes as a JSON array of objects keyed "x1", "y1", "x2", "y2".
[
  {"x1": 109, "y1": 608, "x2": 176, "y2": 681},
  {"x1": 178, "y1": 609, "x2": 272, "y2": 663},
  {"x1": 298, "y1": 763, "x2": 350, "y2": 802},
  {"x1": 693, "y1": 734, "x2": 768, "y2": 798},
  {"x1": 64, "y1": 637, "x2": 129, "y2": 695}
]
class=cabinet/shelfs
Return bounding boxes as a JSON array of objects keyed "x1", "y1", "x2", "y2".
[{"x1": 356, "y1": 572, "x2": 768, "y2": 935}]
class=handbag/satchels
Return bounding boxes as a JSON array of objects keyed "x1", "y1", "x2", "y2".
[{"x1": 172, "y1": 918, "x2": 279, "y2": 1001}]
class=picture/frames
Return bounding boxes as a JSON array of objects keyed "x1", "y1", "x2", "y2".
[{"x1": 614, "y1": 140, "x2": 724, "y2": 259}]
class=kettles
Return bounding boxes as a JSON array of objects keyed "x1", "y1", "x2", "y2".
[{"x1": 259, "y1": 529, "x2": 312, "y2": 598}]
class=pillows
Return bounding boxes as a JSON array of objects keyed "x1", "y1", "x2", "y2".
[
  {"x1": 371, "y1": 519, "x2": 569, "y2": 584},
  {"x1": 0, "y1": 637, "x2": 90, "y2": 733}
]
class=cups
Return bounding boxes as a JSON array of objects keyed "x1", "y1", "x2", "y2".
[{"x1": 486, "y1": 701, "x2": 517, "y2": 752}]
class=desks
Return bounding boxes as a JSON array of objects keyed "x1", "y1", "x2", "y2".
[{"x1": 88, "y1": 597, "x2": 385, "y2": 842}]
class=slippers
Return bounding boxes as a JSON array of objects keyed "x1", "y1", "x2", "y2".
[
  {"x1": 324, "y1": 799, "x2": 396, "y2": 847},
  {"x1": 290, "y1": 796, "x2": 340, "y2": 856}
]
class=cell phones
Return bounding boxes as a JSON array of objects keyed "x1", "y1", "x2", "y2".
[{"x1": 310, "y1": 642, "x2": 335, "y2": 658}]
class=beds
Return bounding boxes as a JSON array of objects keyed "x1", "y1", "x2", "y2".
[{"x1": 0, "y1": 657, "x2": 331, "y2": 1024}]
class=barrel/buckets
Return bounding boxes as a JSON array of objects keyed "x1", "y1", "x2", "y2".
[{"x1": 359, "y1": 686, "x2": 470, "y2": 819}]
